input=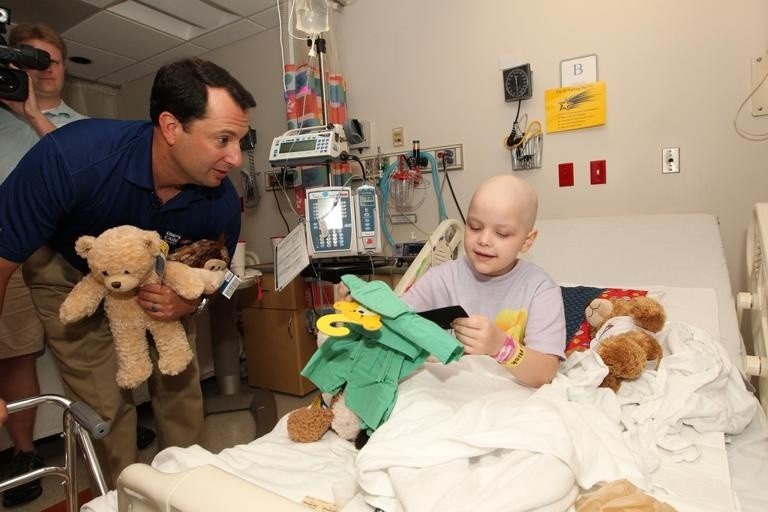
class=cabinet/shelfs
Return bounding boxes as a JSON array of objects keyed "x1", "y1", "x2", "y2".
[{"x1": 240, "y1": 264, "x2": 317, "y2": 397}]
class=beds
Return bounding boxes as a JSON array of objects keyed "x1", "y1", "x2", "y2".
[{"x1": 1, "y1": 214, "x2": 767, "y2": 512}]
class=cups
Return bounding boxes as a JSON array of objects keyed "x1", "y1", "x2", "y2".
[{"x1": 230, "y1": 241, "x2": 248, "y2": 281}]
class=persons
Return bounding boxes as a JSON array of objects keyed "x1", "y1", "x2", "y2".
[
  {"x1": 0, "y1": 22, "x2": 156, "y2": 507},
  {"x1": 0, "y1": 52, "x2": 257, "y2": 491},
  {"x1": 335, "y1": 174, "x2": 567, "y2": 390}
]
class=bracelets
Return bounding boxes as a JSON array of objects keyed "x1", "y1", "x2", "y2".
[
  {"x1": 507, "y1": 343, "x2": 525, "y2": 368},
  {"x1": 497, "y1": 336, "x2": 514, "y2": 365}
]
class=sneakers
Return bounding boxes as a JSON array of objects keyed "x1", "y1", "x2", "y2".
[{"x1": 4, "y1": 446, "x2": 42, "y2": 506}]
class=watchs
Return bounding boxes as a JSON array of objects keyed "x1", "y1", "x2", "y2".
[{"x1": 196, "y1": 296, "x2": 210, "y2": 316}]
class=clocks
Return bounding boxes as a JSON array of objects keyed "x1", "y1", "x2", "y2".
[{"x1": 502, "y1": 64, "x2": 532, "y2": 101}]
